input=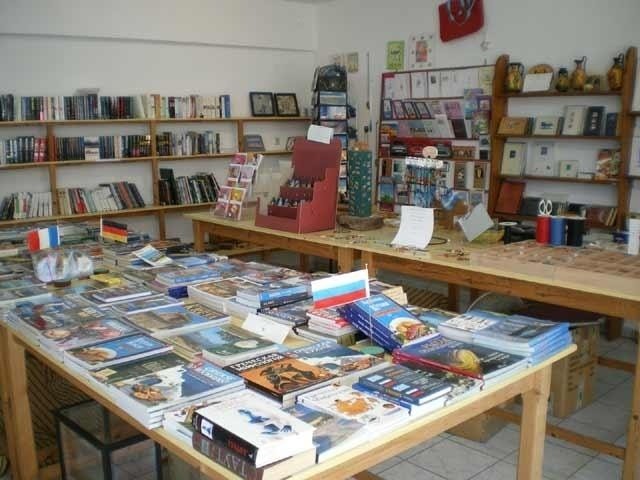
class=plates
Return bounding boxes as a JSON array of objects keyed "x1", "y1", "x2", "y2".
[
  {"x1": 527, "y1": 63, "x2": 554, "y2": 84},
  {"x1": 421, "y1": 146, "x2": 439, "y2": 159}
]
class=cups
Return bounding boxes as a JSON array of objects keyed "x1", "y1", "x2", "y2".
[
  {"x1": 535, "y1": 215, "x2": 551, "y2": 245},
  {"x1": 626, "y1": 217, "x2": 640, "y2": 257},
  {"x1": 566, "y1": 216, "x2": 586, "y2": 247},
  {"x1": 549, "y1": 215, "x2": 566, "y2": 246}
]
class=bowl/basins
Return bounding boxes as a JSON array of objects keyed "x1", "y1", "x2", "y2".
[{"x1": 473, "y1": 227, "x2": 505, "y2": 246}]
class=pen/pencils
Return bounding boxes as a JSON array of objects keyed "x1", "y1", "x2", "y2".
[{"x1": 557, "y1": 199, "x2": 570, "y2": 216}]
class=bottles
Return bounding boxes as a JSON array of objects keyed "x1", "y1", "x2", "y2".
[
  {"x1": 270, "y1": 195, "x2": 299, "y2": 208},
  {"x1": 286, "y1": 177, "x2": 313, "y2": 189},
  {"x1": 555, "y1": 68, "x2": 571, "y2": 92}
]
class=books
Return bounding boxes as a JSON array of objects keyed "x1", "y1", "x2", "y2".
[{"x1": 0, "y1": 93, "x2": 640, "y2": 480}]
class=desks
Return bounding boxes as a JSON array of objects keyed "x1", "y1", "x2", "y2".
[
  {"x1": 182, "y1": 208, "x2": 639, "y2": 480},
  {"x1": 0, "y1": 239, "x2": 577, "y2": 479}
]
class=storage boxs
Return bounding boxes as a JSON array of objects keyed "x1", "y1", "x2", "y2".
[
  {"x1": 546, "y1": 326, "x2": 599, "y2": 419},
  {"x1": 50, "y1": 399, "x2": 163, "y2": 480}
]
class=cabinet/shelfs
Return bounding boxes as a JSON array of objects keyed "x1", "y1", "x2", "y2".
[
  {"x1": 493, "y1": 46, "x2": 637, "y2": 241},
  {"x1": 255, "y1": 136, "x2": 342, "y2": 234},
  {"x1": 375, "y1": 54, "x2": 509, "y2": 217},
  {"x1": 0, "y1": 116, "x2": 313, "y2": 241}
]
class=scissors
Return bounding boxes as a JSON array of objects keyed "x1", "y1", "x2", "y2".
[{"x1": 537, "y1": 200, "x2": 554, "y2": 215}]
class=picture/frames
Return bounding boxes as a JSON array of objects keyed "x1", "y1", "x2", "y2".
[
  {"x1": 409, "y1": 32, "x2": 436, "y2": 70},
  {"x1": 386, "y1": 41, "x2": 406, "y2": 69},
  {"x1": 383, "y1": 98, "x2": 431, "y2": 120},
  {"x1": 249, "y1": 92, "x2": 275, "y2": 116},
  {"x1": 274, "y1": 93, "x2": 299, "y2": 117}
]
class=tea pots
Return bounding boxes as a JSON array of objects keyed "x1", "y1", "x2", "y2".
[
  {"x1": 604, "y1": 52, "x2": 624, "y2": 90},
  {"x1": 569, "y1": 55, "x2": 587, "y2": 91},
  {"x1": 502, "y1": 62, "x2": 524, "y2": 93}
]
